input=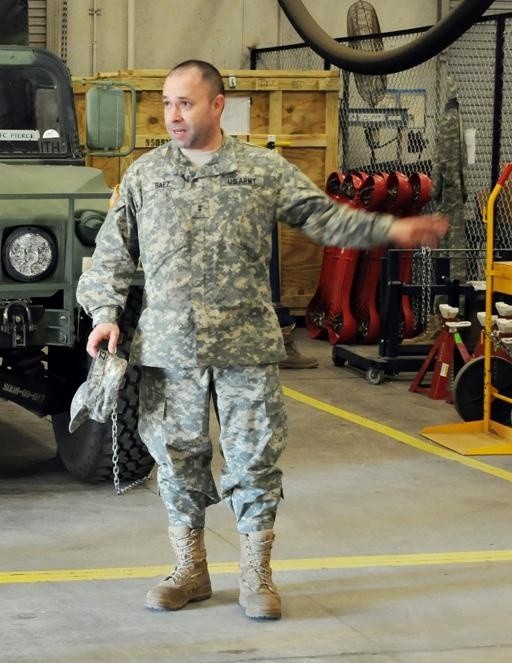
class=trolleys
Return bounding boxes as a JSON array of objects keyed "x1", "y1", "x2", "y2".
[{"x1": 332, "y1": 281, "x2": 476, "y2": 385}]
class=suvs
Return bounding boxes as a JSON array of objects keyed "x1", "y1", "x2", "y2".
[{"x1": 0, "y1": 44, "x2": 155, "y2": 485}]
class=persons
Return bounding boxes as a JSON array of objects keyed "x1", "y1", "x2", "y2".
[{"x1": 75, "y1": 58, "x2": 453, "y2": 621}]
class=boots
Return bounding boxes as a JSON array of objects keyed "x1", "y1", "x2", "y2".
[
  {"x1": 144, "y1": 526, "x2": 212, "y2": 612},
  {"x1": 278, "y1": 323, "x2": 321, "y2": 370},
  {"x1": 237, "y1": 529, "x2": 282, "y2": 621}
]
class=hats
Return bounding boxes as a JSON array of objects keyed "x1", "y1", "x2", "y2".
[{"x1": 68, "y1": 349, "x2": 128, "y2": 434}]
